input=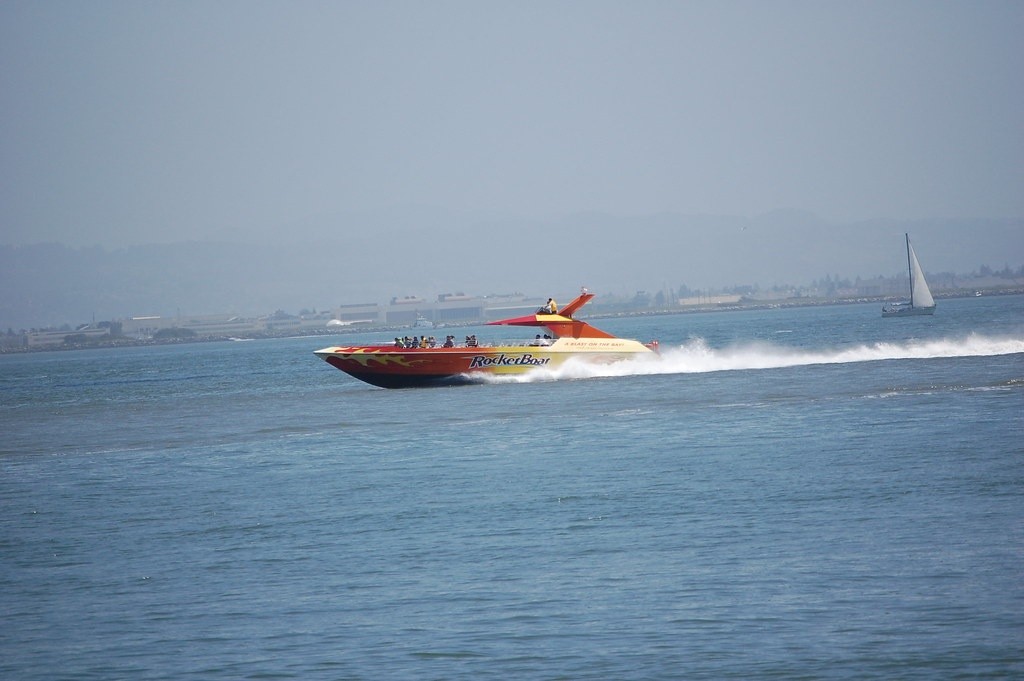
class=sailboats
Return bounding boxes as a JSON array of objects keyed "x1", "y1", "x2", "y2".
[{"x1": 880, "y1": 232, "x2": 936, "y2": 321}]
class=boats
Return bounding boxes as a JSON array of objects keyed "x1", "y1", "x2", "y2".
[{"x1": 313, "y1": 289, "x2": 668, "y2": 391}]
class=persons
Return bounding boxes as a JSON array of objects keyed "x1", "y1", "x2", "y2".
[
  {"x1": 545, "y1": 298, "x2": 557, "y2": 314},
  {"x1": 395, "y1": 336, "x2": 436, "y2": 349},
  {"x1": 530, "y1": 334, "x2": 551, "y2": 346},
  {"x1": 466, "y1": 335, "x2": 476, "y2": 347},
  {"x1": 441, "y1": 335, "x2": 455, "y2": 348}
]
